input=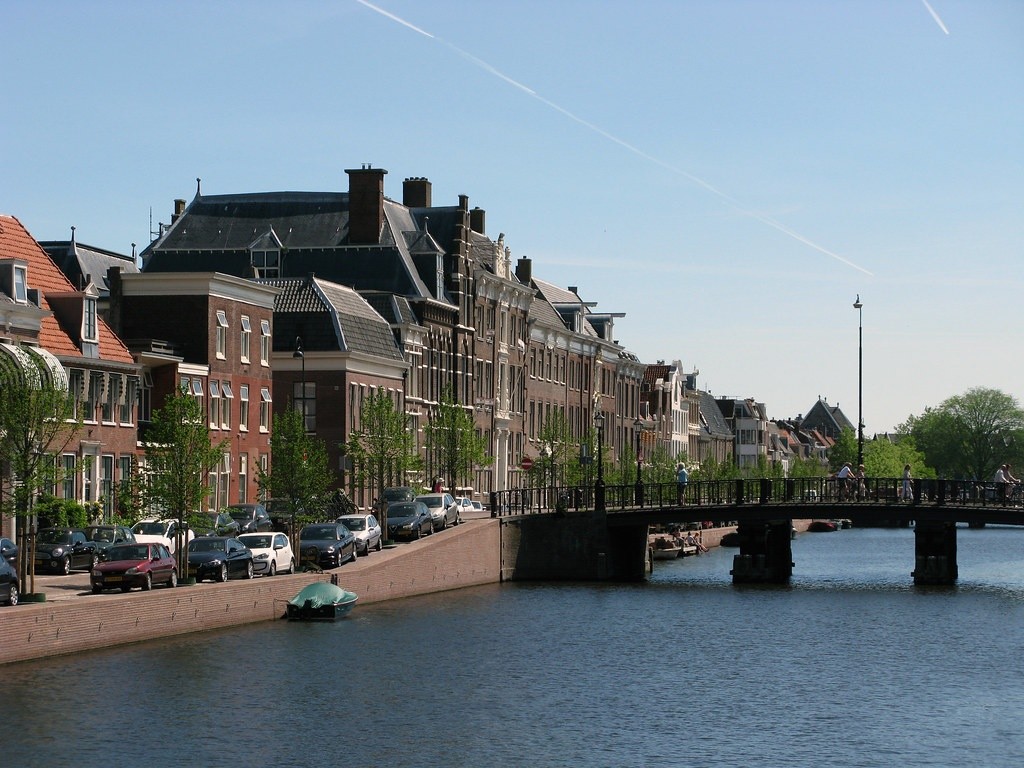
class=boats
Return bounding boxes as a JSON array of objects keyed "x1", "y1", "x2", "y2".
[
  {"x1": 807, "y1": 518, "x2": 852, "y2": 532},
  {"x1": 286, "y1": 583, "x2": 359, "y2": 621},
  {"x1": 790, "y1": 527, "x2": 798, "y2": 540},
  {"x1": 652, "y1": 537, "x2": 699, "y2": 559}
]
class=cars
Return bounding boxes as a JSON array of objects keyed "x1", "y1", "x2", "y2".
[
  {"x1": 127, "y1": 518, "x2": 195, "y2": 554},
  {"x1": 85, "y1": 526, "x2": 138, "y2": 553},
  {"x1": 235, "y1": 531, "x2": 295, "y2": 577},
  {"x1": 410, "y1": 493, "x2": 460, "y2": 531},
  {"x1": 186, "y1": 511, "x2": 241, "y2": 538},
  {"x1": 28, "y1": 526, "x2": 94, "y2": 577},
  {"x1": 90, "y1": 543, "x2": 179, "y2": 594},
  {"x1": 223, "y1": 503, "x2": 274, "y2": 534},
  {"x1": 0, "y1": 553, "x2": 19, "y2": 606},
  {"x1": 334, "y1": 514, "x2": 383, "y2": 556},
  {"x1": 376, "y1": 501, "x2": 434, "y2": 540},
  {"x1": 173, "y1": 535, "x2": 254, "y2": 583},
  {"x1": 261, "y1": 497, "x2": 305, "y2": 536},
  {"x1": 455, "y1": 497, "x2": 475, "y2": 513},
  {"x1": 469, "y1": 501, "x2": 486, "y2": 512},
  {"x1": 294, "y1": 523, "x2": 357, "y2": 569},
  {"x1": 371, "y1": 486, "x2": 417, "y2": 522},
  {"x1": 0, "y1": 536, "x2": 18, "y2": 569}
]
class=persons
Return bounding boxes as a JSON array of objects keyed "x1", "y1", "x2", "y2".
[
  {"x1": 900, "y1": 464, "x2": 914, "y2": 502},
  {"x1": 676, "y1": 462, "x2": 687, "y2": 506},
  {"x1": 1004, "y1": 464, "x2": 1022, "y2": 496},
  {"x1": 686, "y1": 531, "x2": 709, "y2": 553},
  {"x1": 995, "y1": 464, "x2": 1009, "y2": 506},
  {"x1": 434, "y1": 477, "x2": 444, "y2": 493},
  {"x1": 858, "y1": 464, "x2": 874, "y2": 496},
  {"x1": 838, "y1": 462, "x2": 856, "y2": 493}
]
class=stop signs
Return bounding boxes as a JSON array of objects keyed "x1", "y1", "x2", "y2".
[{"x1": 522, "y1": 457, "x2": 532, "y2": 470}]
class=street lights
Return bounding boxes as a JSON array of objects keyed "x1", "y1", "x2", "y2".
[
  {"x1": 851, "y1": 292, "x2": 864, "y2": 469},
  {"x1": 631, "y1": 416, "x2": 644, "y2": 505},
  {"x1": 592, "y1": 412, "x2": 606, "y2": 511},
  {"x1": 291, "y1": 336, "x2": 306, "y2": 441}
]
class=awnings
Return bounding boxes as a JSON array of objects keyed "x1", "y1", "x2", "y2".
[{"x1": 0, "y1": 342, "x2": 69, "y2": 392}]
